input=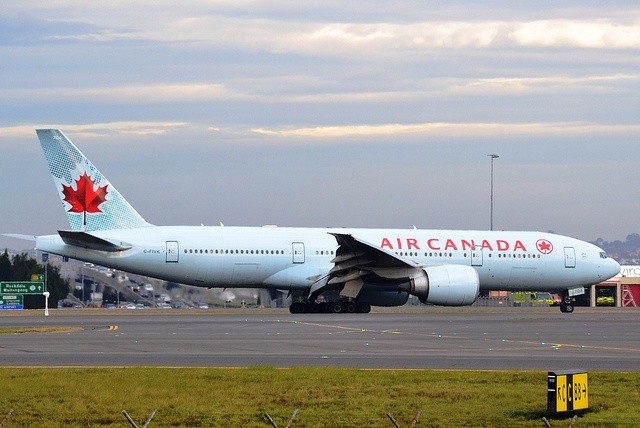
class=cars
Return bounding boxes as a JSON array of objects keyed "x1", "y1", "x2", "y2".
[
  {"x1": 163, "y1": 304, "x2": 171, "y2": 309},
  {"x1": 140, "y1": 291, "x2": 148, "y2": 296},
  {"x1": 144, "y1": 284, "x2": 153, "y2": 290},
  {"x1": 63, "y1": 296, "x2": 73, "y2": 311},
  {"x1": 126, "y1": 304, "x2": 136, "y2": 308},
  {"x1": 199, "y1": 302, "x2": 208, "y2": 308}
]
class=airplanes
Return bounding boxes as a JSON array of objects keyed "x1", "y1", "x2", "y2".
[{"x1": 0, "y1": 128, "x2": 621, "y2": 313}]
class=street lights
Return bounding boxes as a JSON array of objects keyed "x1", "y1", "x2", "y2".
[{"x1": 489, "y1": 154, "x2": 500, "y2": 230}]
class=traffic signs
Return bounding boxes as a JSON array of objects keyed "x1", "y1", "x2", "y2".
[
  {"x1": 0, "y1": 296, "x2": 24, "y2": 310},
  {"x1": 31, "y1": 274, "x2": 45, "y2": 282},
  {"x1": 0, "y1": 281, "x2": 44, "y2": 296}
]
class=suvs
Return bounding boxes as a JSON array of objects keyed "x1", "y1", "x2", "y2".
[
  {"x1": 552, "y1": 294, "x2": 562, "y2": 306},
  {"x1": 597, "y1": 288, "x2": 615, "y2": 306}
]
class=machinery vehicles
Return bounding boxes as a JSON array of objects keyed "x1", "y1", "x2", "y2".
[{"x1": 508, "y1": 291, "x2": 557, "y2": 306}]
